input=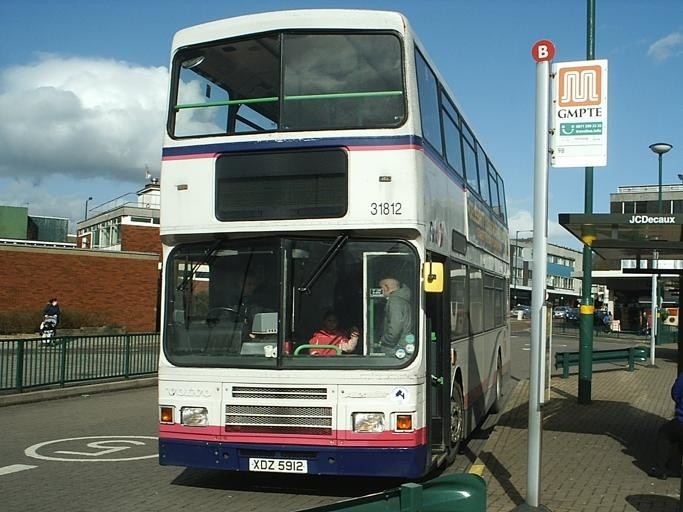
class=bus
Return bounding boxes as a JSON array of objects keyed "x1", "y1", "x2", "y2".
[{"x1": 152, "y1": 4, "x2": 515, "y2": 490}]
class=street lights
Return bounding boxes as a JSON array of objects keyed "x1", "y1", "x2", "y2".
[
  {"x1": 84, "y1": 196, "x2": 93, "y2": 221},
  {"x1": 645, "y1": 141, "x2": 673, "y2": 341}
]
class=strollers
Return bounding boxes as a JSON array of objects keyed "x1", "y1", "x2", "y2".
[{"x1": 36, "y1": 314, "x2": 58, "y2": 347}]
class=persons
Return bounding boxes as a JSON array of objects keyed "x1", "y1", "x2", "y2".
[
  {"x1": 375, "y1": 276, "x2": 416, "y2": 354},
  {"x1": 594, "y1": 311, "x2": 612, "y2": 329},
  {"x1": 229, "y1": 273, "x2": 274, "y2": 328},
  {"x1": 308, "y1": 312, "x2": 360, "y2": 357},
  {"x1": 42, "y1": 298, "x2": 60, "y2": 336},
  {"x1": 647, "y1": 370, "x2": 683, "y2": 480}
]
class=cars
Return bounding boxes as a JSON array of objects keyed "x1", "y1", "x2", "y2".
[{"x1": 509, "y1": 302, "x2": 605, "y2": 323}]
